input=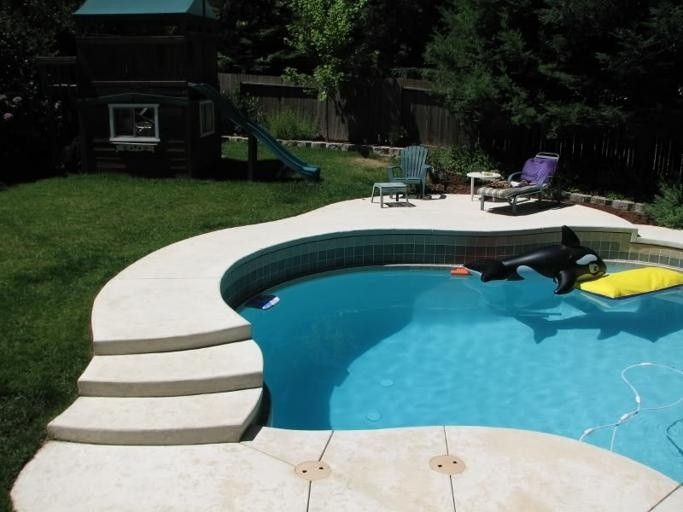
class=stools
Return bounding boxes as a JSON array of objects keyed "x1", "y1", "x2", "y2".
[{"x1": 370, "y1": 181, "x2": 409, "y2": 208}]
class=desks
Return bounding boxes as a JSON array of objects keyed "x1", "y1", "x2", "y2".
[{"x1": 466, "y1": 172, "x2": 501, "y2": 202}]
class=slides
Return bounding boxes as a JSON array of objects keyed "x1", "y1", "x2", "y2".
[{"x1": 192, "y1": 82, "x2": 320, "y2": 183}]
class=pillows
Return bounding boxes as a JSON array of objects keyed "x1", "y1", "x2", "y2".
[{"x1": 520, "y1": 157, "x2": 557, "y2": 183}]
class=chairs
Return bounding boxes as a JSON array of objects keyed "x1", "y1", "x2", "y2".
[
  {"x1": 476, "y1": 151, "x2": 560, "y2": 214},
  {"x1": 387, "y1": 145, "x2": 433, "y2": 199}
]
